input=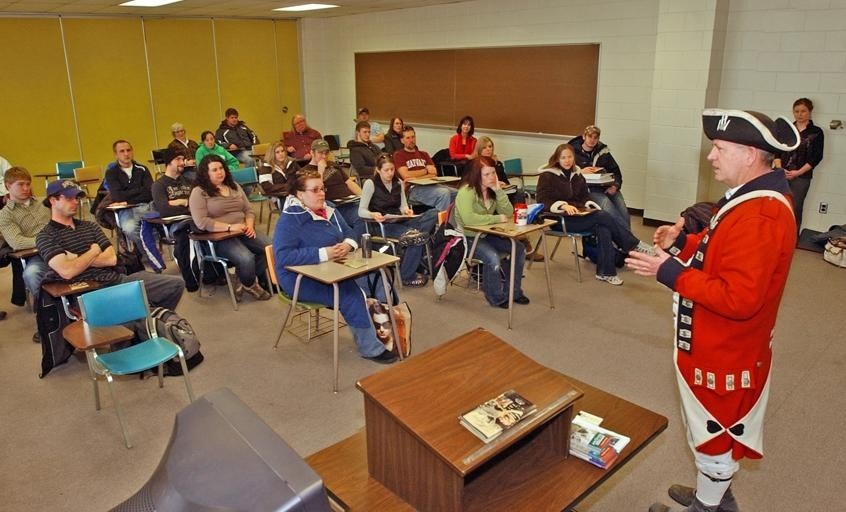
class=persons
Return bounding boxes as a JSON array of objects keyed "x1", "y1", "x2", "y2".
[
  {"x1": 271, "y1": 166, "x2": 401, "y2": 365},
  {"x1": 213, "y1": 108, "x2": 260, "y2": 168},
  {"x1": 567, "y1": 124, "x2": 630, "y2": 237},
  {"x1": 0, "y1": 154, "x2": 14, "y2": 210},
  {"x1": 0, "y1": 166, "x2": 77, "y2": 344},
  {"x1": 448, "y1": 115, "x2": 510, "y2": 189},
  {"x1": 368, "y1": 304, "x2": 404, "y2": 356},
  {"x1": 299, "y1": 139, "x2": 366, "y2": 248},
  {"x1": 537, "y1": 144, "x2": 656, "y2": 286},
  {"x1": 772, "y1": 96, "x2": 824, "y2": 234},
  {"x1": 348, "y1": 105, "x2": 460, "y2": 288},
  {"x1": 103, "y1": 114, "x2": 365, "y2": 302},
  {"x1": 454, "y1": 156, "x2": 534, "y2": 308},
  {"x1": 623, "y1": 109, "x2": 803, "y2": 512},
  {"x1": 34, "y1": 179, "x2": 186, "y2": 376}
]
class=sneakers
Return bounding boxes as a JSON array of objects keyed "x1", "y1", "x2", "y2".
[
  {"x1": 595, "y1": 274, "x2": 624, "y2": 285},
  {"x1": 242, "y1": 274, "x2": 270, "y2": 300},
  {"x1": 361, "y1": 350, "x2": 398, "y2": 364},
  {"x1": 32, "y1": 332, "x2": 40, "y2": 342},
  {"x1": 398, "y1": 229, "x2": 430, "y2": 248},
  {"x1": 402, "y1": 276, "x2": 427, "y2": 288},
  {"x1": 632, "y1": 240, "x2": 656, "y2": 256},
  {"x1": 499, "y1": 301, "x2": 509, "y2": 309},
  {"x1": 514, "y1": 295, "x2": 529, "y2": 305},
  {"x1": 230, "y1": 275, "x2": 243, "y2": 302}
]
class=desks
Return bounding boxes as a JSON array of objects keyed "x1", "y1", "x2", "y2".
[
  {"x1": 0, "y1": 174, "x2": 134, "y2": 411},
  {"x1": 587, "y1": 182, "x2": 612, "y2": 194}
]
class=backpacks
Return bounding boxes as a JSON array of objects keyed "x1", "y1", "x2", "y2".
[{"x1": 133, "y1": 305, "x2": 204, "y2": 376}]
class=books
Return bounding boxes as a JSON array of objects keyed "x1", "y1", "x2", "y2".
[
  {"x1": 457, "y1": 386, "x2": 540, "y2": 443},
  {"x1": 568, "y1": 412, "x2": 630, "y2": 470}
]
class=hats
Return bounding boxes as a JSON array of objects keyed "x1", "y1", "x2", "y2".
[
  {"x1": 702, "y1": 108, "x2": 801, "y2": 156},
  {"x1": 311, "y1": 138, "x2": 330, "y2": 152},
  {"x1": 584, "y1": 125, "x2": 600, "y2": 137},
  {"x1": 46, "y1": 180, "x2": 86, "y2": 198},
  {"x1": 164, "y1": 146, "x2": 182, "y2": 164},
  {"x1": 358, "y1": 107, "x2": 369, "y2": 115}
]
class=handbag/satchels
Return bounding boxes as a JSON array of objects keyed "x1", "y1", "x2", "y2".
[
  {"x1": 367, "y1": 298, "x2": 412, "y2": 357},
  {"x1": 431, "y1": 223, "x2": 467, "y2": 284},
  {"x1": 582, "y1": 234, "x2": 630, "y2": 268},
  {"x1": 823, "y1": 236, "x2": 846, "y2": 269},
  {"x1": 325, "y1": 135, "x2": 339, "y2": 150},
  {"x1": 117, "y1": 252, "x2": 145, "y2": 275}
]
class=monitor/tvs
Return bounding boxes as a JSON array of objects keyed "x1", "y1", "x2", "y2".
[{"x1": 108, "y1": 388, "x2": 332, "y2": 512}]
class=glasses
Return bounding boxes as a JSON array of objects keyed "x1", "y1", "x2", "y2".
[
  {"x1": 379, "y1": 154, "x2": 393, "y2": 159},
  {"x1": 297, "y1": 187, "x2": 327, "y2": 193}
]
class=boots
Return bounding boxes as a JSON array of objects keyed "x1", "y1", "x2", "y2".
[
  {"x1": 650, "y1": 484, "x2": 722, "y2": 512},
  {"x1": 525, "y1": 241, "x2": 545, "y2": 262}
]
